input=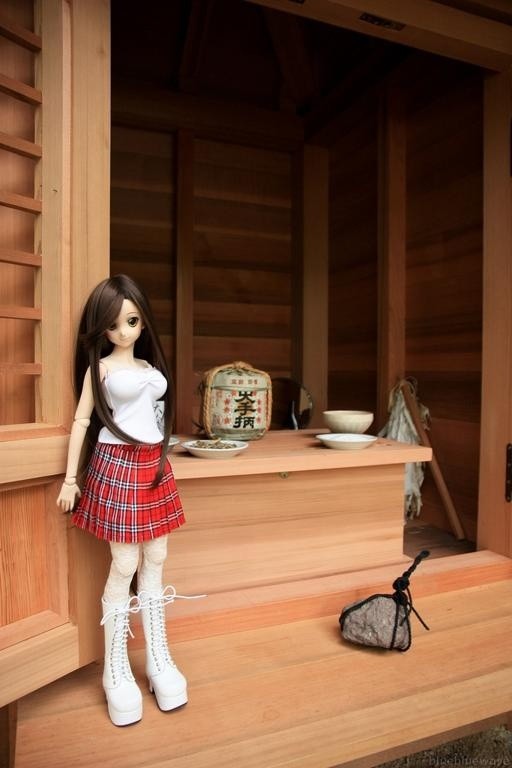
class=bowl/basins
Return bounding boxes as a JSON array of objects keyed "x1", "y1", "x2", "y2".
[{"x1": 322, "y1": 410, "x2": 375, "y2": 433}]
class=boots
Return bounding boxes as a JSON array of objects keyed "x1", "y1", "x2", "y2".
[
  {"x1": 136, "y1": 588, "x2": 190, "y2": 714},
  {"x1": 98, "y1": 595, "x2": 144, "y2": 729}
]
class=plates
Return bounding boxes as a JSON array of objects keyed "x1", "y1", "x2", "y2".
[
  {"x1": 182, "y1": 440, "x2": 248, "y2": 458},
  {"x1": 316, "y1": 432, "x2": 378, "y2": 450}
]
